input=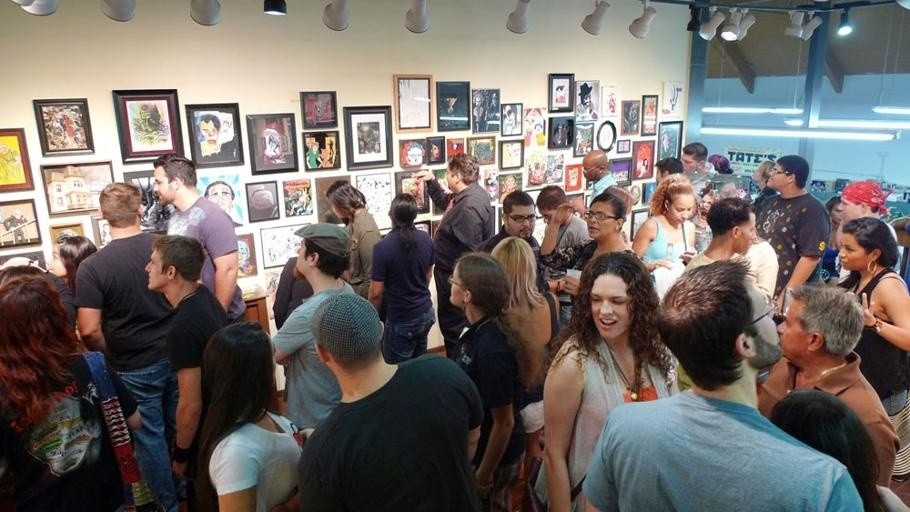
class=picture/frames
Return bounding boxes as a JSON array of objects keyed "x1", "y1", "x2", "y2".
[
  {"x1": 123, "y1": 170, "x2": 175, "y2": 235},
  {"x1": 602, "y1": 85, "x2": 621, "y2": 117},
  {"x1": 658, "y1": 121, "x2": 683, "y2": 162},
  {"x1": 499, "y1": 207, "x2": 504, "y2": 231},
  {"x1": 303, "y1": 131, "x2": 341, "y2": 171},
  {"x1": 467, "y1": 136, "x2": 495, "y2": 165},
  {"x1": 0, "y1": 199, "x2": 42, "y2": 249},
  {"x1": 414, "y1": 220, "x2": 431, "y2": 237},
  {"x1": 344, "y1": 105, "x2": 393, "y2": 171},
  {"x1": 470, "y1": 88, "x2": 501, "y2": 135},
  {"x1": 427, "y1": 136, "x2": 445, "y2": 165},
  {"x1": 586, "y1": 180, "x2": 597, "y2": 190},
  {"x1": 49, "y1": 223, "x2": 84, "y2": 244},
  {"x1": 630, "y1": 186, "x2": 641, "y2": 205},
  {"x1": 91, "y1": 212, "x2": 112, "y2": 249},
  {"x1": 394, "y1": 74, "x2": 435, "y2": 134},
  {"x1": 524, "y1": 189, "x2": 543, "y2": 219},
  {"x1": 260, "y1": 224, "x2": 311, "y2": 268},
  {"x1": 112, "y1": 89, "x2": 185, "y2": 165},
  {"x1": 437, "y1": 81, "x2": 470, "y2": 131},
  {"x1": 356, "y1": 173, "x2": 393, "y2": 230},
  {"x1": 609, "y1": 157, "x2": 632, "y2": 186},
  {"x1": 573, "y1": 123, "x2": 594, "y2": 157},
  {"x1": 301, "y1": 91, "x2": 338, "y2": 129},
  {"x1": 395, "y1": 169, "x2": 430, "y2": 214},
  {"x1": 641, "y1": 95, "x2": 658, "y2": 136},
  {"x1": 33, "y1": 98, "x2": 95, "y2": 157},
  {"x1": 621, "y1": 101, "x2": 639, "y2": 135},
  {"x1": 501, "y1": 103, "x2": 523, "y2": 136},
  {"x1": 0, "y1": 128, "x2": 35, "y2": 192},
  {"x1": 567, "y1": 193, "x2": 584, "y2": 210},
  {"x1": 632, "y1": 140, "x2": 655, "y2": 179},
  {"x1": 565, "y1": 164, "x2": 582, "y2": 192},
  {"x1": 246, "y1": 113, "x2": 298, "y2": 176},
  {"x1": 185, "y1": 103, "x2": 244, "y2": 167},
  {"x1": 585, "y1": 195, "x2": 591, "y2": 208},
  {"x1": 643, "y1": 181, "x2": 657, "y2": 203},
  {"x1": 500, "y1": 140, "x2": 524, "y2": 169},
  {"x1": 597, "y1": 121, "x2": 617, "y2": 152},
  {"x1": 39, "y1": 162, "x2": 114, "y2": 216},
  {"x1": 630, "y1": 208, "x2": 648, "y2": 241},
  {"x1": 617, "y1": 140, "x2": 630, "y2": 153},
  {"x1": 548, "y1": 73, "x2": 574, "y2": 112},
  {"x1": 575, "y1": 80, "x2": 601, "y2": 123},
  {"x1": 245, "y1": 181, "x2": 280, "y2": 223}
]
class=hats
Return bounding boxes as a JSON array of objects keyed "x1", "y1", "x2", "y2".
[
  {"x1": 578, "y1": 83, "x2": 593, "y2": 95},
  {"x1": 293, "y1": 222, "x2": 354, "y2": 258},
  {"x1": 308, "y1": 291, "x2": 382, "y2": 362}
]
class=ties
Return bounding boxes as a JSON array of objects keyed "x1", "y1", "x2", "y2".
[{"x1": 431, "y1": 195, "x2": 455, "y2": 240}]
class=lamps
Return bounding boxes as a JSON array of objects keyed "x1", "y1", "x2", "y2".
[
  {"x1": 629, "y1": 0, "x2": 656, "y2": 39},
  {"x1": 20, "y1": 0, "x2": 59, "y2": 16},
  {"x1": 190, "y1": 0, "x2": 221, "y2": 26},
  {"x1": 737, "y1": 8, "x2": 756, "y2": 41},
  {"x1": 721, "y1": 8, "x2": 742, "y2": 41},
  {"x1": 687, "y1": 4, "x2": 702, "y2": 32},
  {"x1": 582, "y1": 0, "x2": 610, "y2": 36},
  {"x1": 699, "y1": 6, "x2": 726, "y2": 41},
  {"x1": 838, "y1": 8, "x2": 852, "y2": 36},
  {"x1": 405, "y1": 0, "x2": 431, "y2": 33},
  {"x1": 783, "y1": 10, "x2": 805, "y2": 38},
  {"x1": 322, "y1": 0, "x2": 352, "y2": 32},
  {"x1": 802, "y1": 10, "x2": 823, "y2": 41},
  {"x1": 506, "y1": 0, "x2": 530, "y2": 34},
  {"x1": 100, "y1": 0, "x2": 136, "y2": 21},
  {"x1": 11, "y1": 0, "x2": 34, "y2": 6},
  {"x1": 264, "y1": 0, "x2": 286, "y2": 16}
]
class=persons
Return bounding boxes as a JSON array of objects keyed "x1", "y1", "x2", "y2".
[
  {"x1": 504, "y1": 106, "x2": 522, "y2": 135},
  {"x1": 2, "y1": 144, "x2": 910, "y2": 512},
  {"x1": 472, "y1": 92, "x2": 485, "y2": 132},
  {"x1": 579, "y1": 83, "x2": 596, "y2": 120}
]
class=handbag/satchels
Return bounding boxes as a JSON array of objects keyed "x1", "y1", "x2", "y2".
[
  {"x1": 491, "y1": 452, "x2": 548, "y2": 512},
  {"x1": 116, "y1": 474, "x2": 171, "y2": 512}
]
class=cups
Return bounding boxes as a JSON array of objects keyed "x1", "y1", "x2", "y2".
[{"x1": 567, "y1": 269, "x2": 582, "y2": 280}]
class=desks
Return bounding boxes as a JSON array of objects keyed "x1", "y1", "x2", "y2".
[{"x1": 243, "y1": 284, "x2": 279, "y2": 413}]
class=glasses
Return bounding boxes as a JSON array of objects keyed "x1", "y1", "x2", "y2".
[
  {"x1": 770, "y1": 166, "x2": 791, "y2": 176},
  {"x1": 748, "y1": 284, "x2": 777, "y2": 326},
  {"x1": 509, "y1": 213, "x2": 536, "y2": 223},
  {"x1": 447, "y1": 274, "x2": 466, "y2": 292},
  {"x1": 584, "y1": 209, "x2": 619, "y2": 221}
]
